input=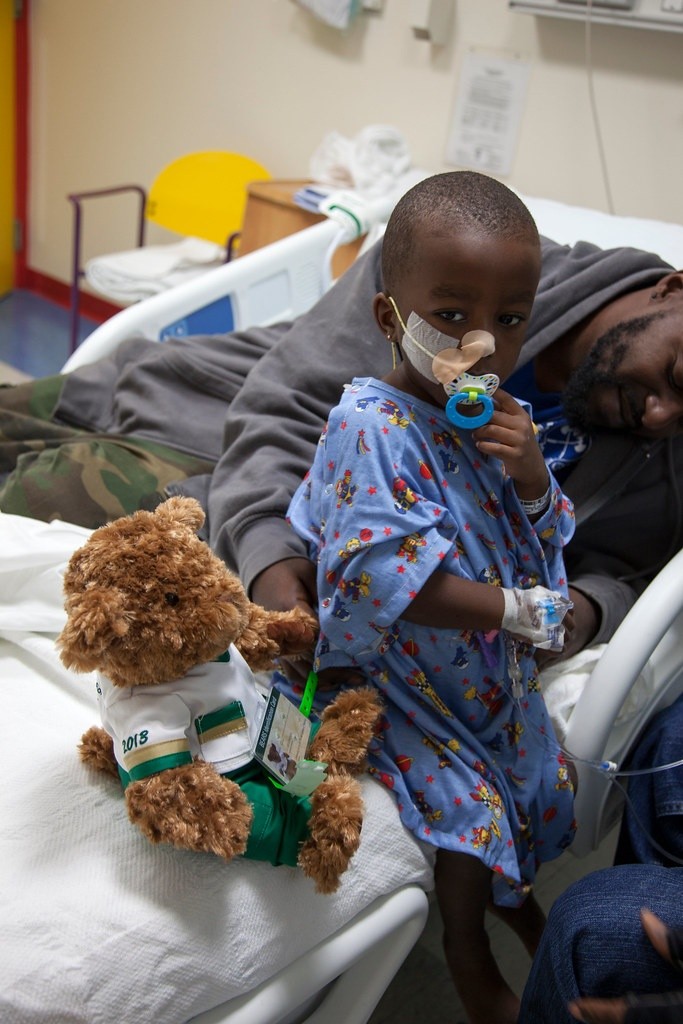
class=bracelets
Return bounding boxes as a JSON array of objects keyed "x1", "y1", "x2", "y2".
[{"x1": 517, "y1": 486, "x2": 552, "y2": 518}]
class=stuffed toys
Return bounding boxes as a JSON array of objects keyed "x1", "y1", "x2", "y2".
[{"x1": 55, "y1": 494, "x2": 385, "y2": 899}]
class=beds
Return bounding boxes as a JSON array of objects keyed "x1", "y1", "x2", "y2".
[{"x1": 0, "y1": 186, "x2": 683, "y2": 1024}]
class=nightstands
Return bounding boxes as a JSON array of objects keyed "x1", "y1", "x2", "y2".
[{"x1": 236, "y1": 178, "x2": 375, "y2": 285}]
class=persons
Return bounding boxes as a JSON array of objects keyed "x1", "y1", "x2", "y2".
[
  {"x1": 279, "y1": 167, "x2": 582, "y2": 1022},
  {"x1": 3, "y1": 226, "x2": 683, "y2": 637},
  {"x1": 518, "y1": 691, "x2": 683, "y2": 1024}
]
